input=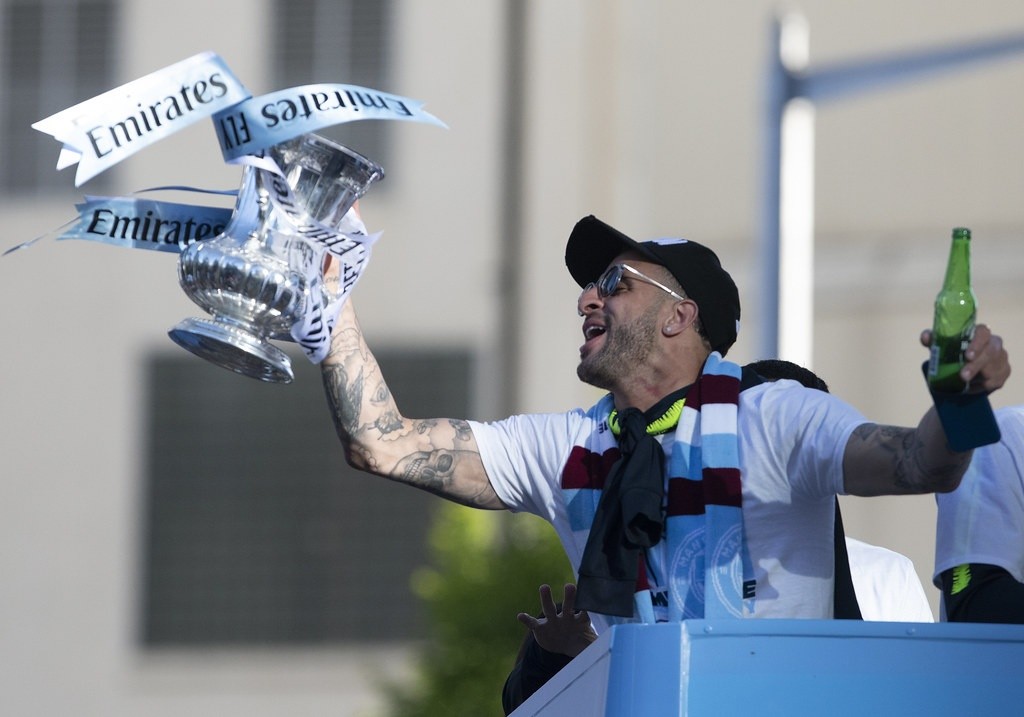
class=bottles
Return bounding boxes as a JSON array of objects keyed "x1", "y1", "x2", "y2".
[{"x1": 927, "y1": 227, "x2": 977, "y2": 397}]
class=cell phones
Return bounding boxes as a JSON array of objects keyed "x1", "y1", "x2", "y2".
[{"x1": 922, "y1": 359, "x2": 1002, "y2": 451}]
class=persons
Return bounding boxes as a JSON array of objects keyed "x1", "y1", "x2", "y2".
[
  {"x1": 314, "y1": 195, "x2": 1012, "y2": 637},
  {"x1": 501, "y1": 358, "x2": 935, "y2": 717},
  {"x1": 930, "y1": 401, "x2": 1024, "y2": 623}
]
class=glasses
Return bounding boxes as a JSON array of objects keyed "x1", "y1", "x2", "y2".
[{"x1": 578, "y1": 264, "x2": 699, "y2": 332}]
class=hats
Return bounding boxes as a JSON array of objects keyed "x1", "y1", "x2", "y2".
[{"x1": 565, "y1": 215, "x2": 741, "y2": 358}]
